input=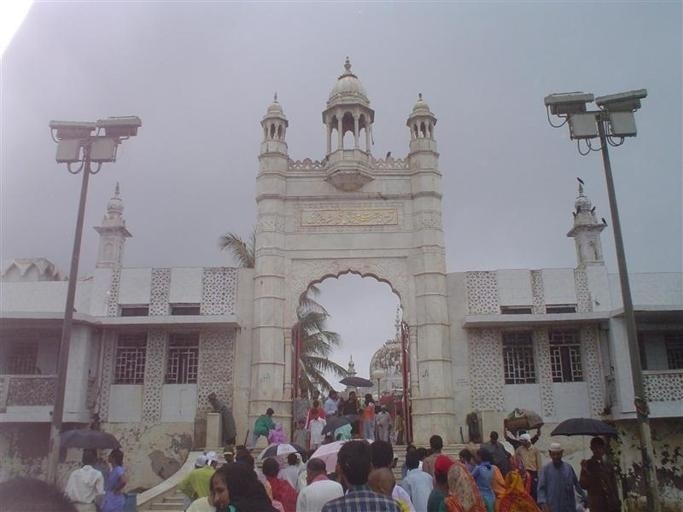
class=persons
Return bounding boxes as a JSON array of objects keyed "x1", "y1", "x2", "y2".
[
  {"x1": 537, "y1": 443, "x2": 587, "y2": 512},
  {"x1": 209, "y1": 392, "x2": 237, "y2": 445},
  {"x1": 64, "y1": 448, "x2": 128, "y2": 512},
  {"x1": 0, "y1": 476, "x2": 79, "y2": 512},
  {"x1": 253, "y1": 407, "x2": 277, "y2": 439},
  {"x1": 182, "y1": 429, "x2": 544, "y2": 512},
  {"x1": 292, "y1": 390, "x2": 404, "y2": 454},
  {"x1": 579, "y1": 437, "x2": 622, "y2": 512}
]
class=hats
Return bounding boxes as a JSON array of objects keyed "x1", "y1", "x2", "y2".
[{"x1": 548, "y1": 443, "x2": 561, "y2": 452}]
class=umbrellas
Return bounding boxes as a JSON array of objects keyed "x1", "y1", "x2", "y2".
[
  {"x1": 550, "y1": 417, "x2": 620, "y2": 459},
  {"x1": 339, "y1": 377, "x2": 375, "y2": 394},
  {"x1": 61, "y1": 428, "x2": 121, "y2": 450}
]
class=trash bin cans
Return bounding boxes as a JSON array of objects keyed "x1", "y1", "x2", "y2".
[{"x1": 465, "y1": 411, "x2": 483, "y2": 444}]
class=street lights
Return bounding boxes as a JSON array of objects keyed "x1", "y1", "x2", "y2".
[
  {"x1": 40, "y1": 114, "x2": 147, "y2": 484},
  {"x1": 372, "y1": 370, "x2": 384, "y2": 401},
  {"x1": 537, "y1": 87, "x2": 657, "y2": 504}
]
